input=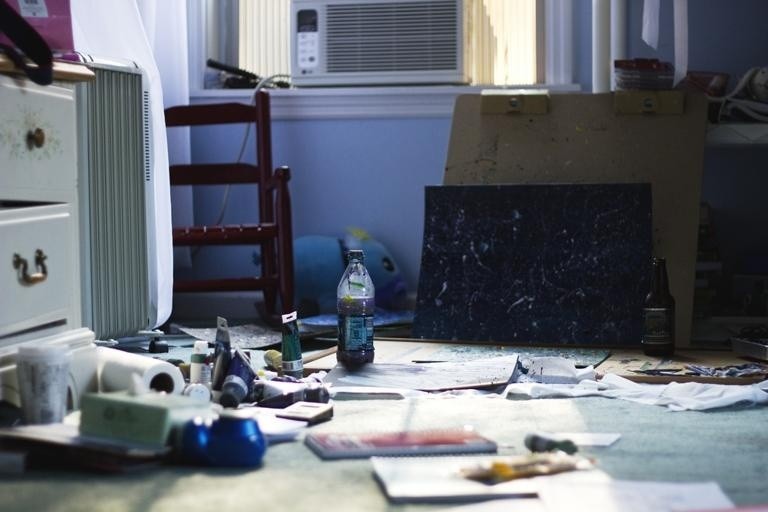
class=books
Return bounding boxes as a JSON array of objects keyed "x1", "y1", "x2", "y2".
[
  {"x1": 414, "y1": 354, "x2": 522, "y2": 391},
  {"x1": 322, "y1": 360, "x2": 423, "y2": 399},
  {"x1": 304, "y1": 423, "x2": 498, "y2": 459},
  {"x1": 371, "y1": 451, "x2": 612, "y2": 502}
]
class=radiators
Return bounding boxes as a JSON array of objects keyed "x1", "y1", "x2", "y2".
[{"x1": 66, "y1": 52, "x2": 159, "y2": 349}]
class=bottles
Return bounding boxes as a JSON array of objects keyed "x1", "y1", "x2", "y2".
[
  {"x1": 335, "y1": 250, "x2": 376, "y2": 367},
  {"x1": 642, "y1": 261, "x2": 676, "y2": 358}
]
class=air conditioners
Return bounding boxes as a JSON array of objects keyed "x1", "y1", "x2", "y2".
[{"x1": 287, "y1": 0, "x2": 497, "y2": 88}]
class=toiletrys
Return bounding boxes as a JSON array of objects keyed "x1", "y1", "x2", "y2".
[
  {"x1": 208, "y1": 317, "x2": 234, "y2": 403},
  {"x1": 189, "y1": 340, "x2": 211, "y2": 390},
  {"x1": 221, "y1": 348, "x2": 260, "y2": 407},
  {"x1": 280, "y1": 308, "x2": 305, "y2": 379}
]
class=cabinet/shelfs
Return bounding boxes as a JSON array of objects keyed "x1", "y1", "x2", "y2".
[{"x1": 0, "y1": 57, "x2": 99, "y2": 424}]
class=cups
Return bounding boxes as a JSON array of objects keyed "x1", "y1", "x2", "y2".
[{"x1": 15, "y1": 344, "x2": 70, "y2": 423}]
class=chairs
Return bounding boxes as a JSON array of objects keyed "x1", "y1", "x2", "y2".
[{"x1": 163, "y1": 89, "x2": 298, "y2": 331}]
